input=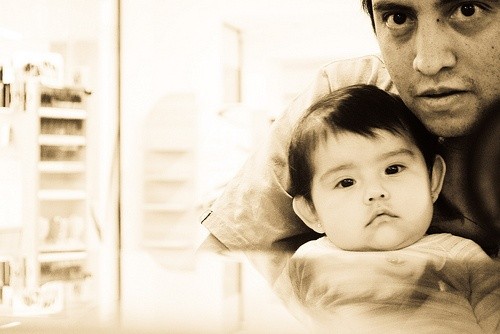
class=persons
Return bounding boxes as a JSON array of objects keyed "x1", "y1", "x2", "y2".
[
  {"x1": 190, "y1": 0, "x2": 499, "y2": 252},
  {"x1": 278, "y1": 83, "x2": 499, "y2": 333}
]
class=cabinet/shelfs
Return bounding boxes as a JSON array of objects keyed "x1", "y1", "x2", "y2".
[{"x1": 0, "y1": 49, "x2": 115, "y2": 322}]
